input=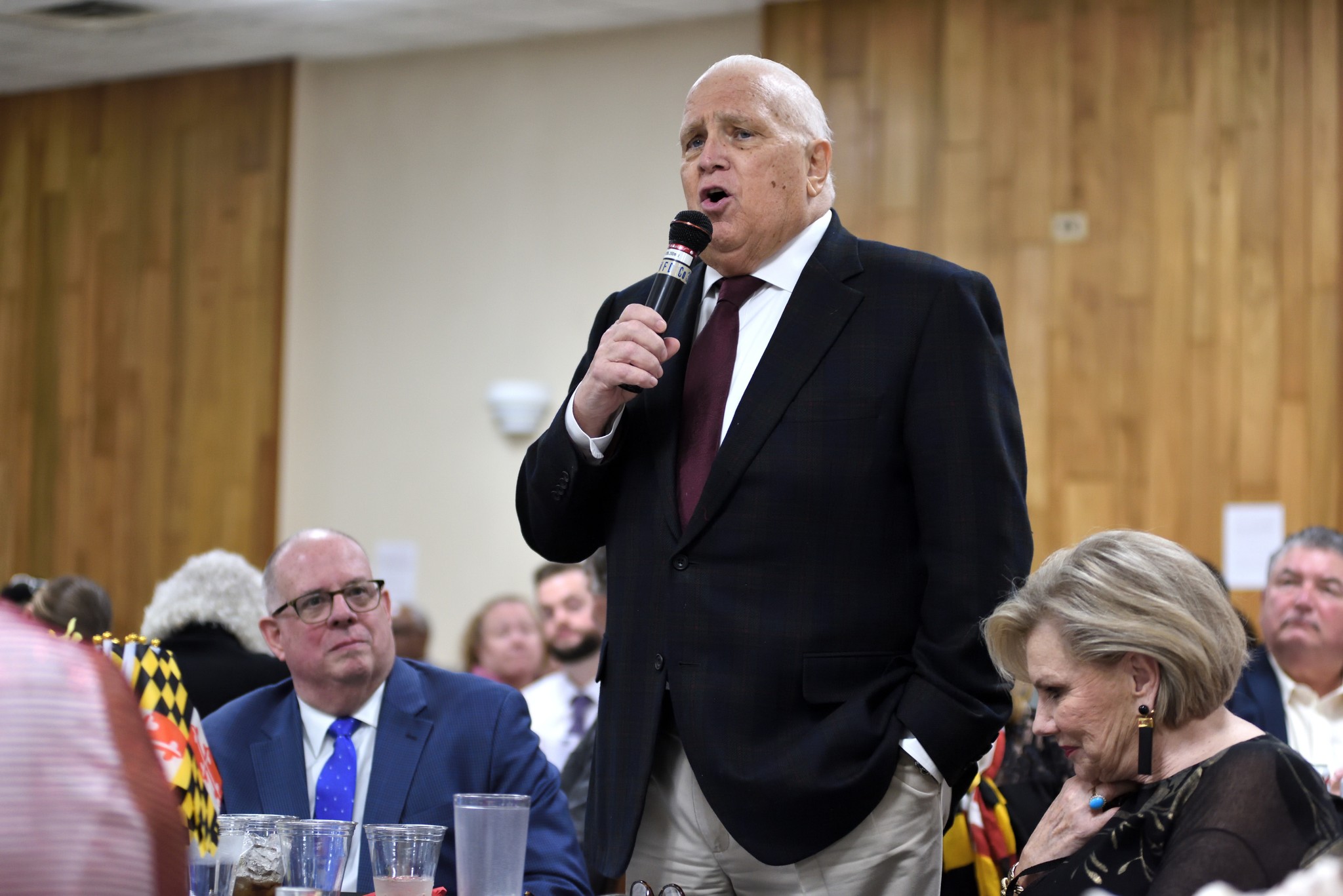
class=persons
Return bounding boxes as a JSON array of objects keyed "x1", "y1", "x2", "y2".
[
  {"x1": 979, "y1": 527, "x2": 1343, "y2": 896},
  {"x1": 140, "y1": 548, "x2": 293, "y2": 720},
  {"x1": 464, "y1": 595, "x2": 553, "y2": 692},
  {"x1": 199, "y1": 526, "x2": 592, "y2": 896},
  {"x1": 23, "y1": 574, "x2": 112, "y2": 646},
  {"x1": 387, "y1": 602, "x2": 431, "y2": 662},
  {"x1": 521, "y1": 558, "x2": 604, "y2": 770},
  {"x1": 515, "y1": 54, "x2": 1034, "y2": 896}
]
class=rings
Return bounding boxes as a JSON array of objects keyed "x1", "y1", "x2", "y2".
[{"x1": 1088, "y1": 787, "x2": 1106, "y2": 810}]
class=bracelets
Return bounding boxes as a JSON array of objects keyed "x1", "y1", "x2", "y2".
[
  {"x1": 1001, "y1": 890, "x2": 1006, "y2": 896},
  {"x1": 1007, "y1": 862, "x2": 1024, "y2": 894},
  {"x1": 1000, "y1": 878, "x2": 1018, "y2": 896}
]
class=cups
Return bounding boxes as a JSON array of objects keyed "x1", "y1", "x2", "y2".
[
  {"x1": 274, "y1": 819, "x2": 358, "y2": 896},
  {"x1": 363, "y1": 824, "x2": 449, "y2": 896},
  {"x1": 453, "y1": 794, "x2": 530, "y2": 896},
  {"x1": 219, "y1": 814, "x2": 299, "y2": 896},
  {"x1": 188, "y1": 817, "x2": 250, "y2": 896}
]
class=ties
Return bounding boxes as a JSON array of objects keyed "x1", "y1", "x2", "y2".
[
  {"x1": 310, "y1": 718, "x2": 364, "y2": 891},
  {"x1": 568, "y1": 697, "x2": 595, "y2": 739},
  {"x1": 676, "y1": 273, "x2": 766, "y2": 524}
]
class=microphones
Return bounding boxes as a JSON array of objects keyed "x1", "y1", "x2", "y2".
[{"x1": 617, "y1": 211, "x2": 714, "y2": 394}]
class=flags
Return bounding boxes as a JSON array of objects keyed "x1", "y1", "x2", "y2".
[{"x1": 95, "y1": 634, "x2": 224, "y2": 853}]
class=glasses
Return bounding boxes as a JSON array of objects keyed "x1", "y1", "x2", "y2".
[{"x1": 271, "y1": 579, "x2": 385, "y2": 624}]
